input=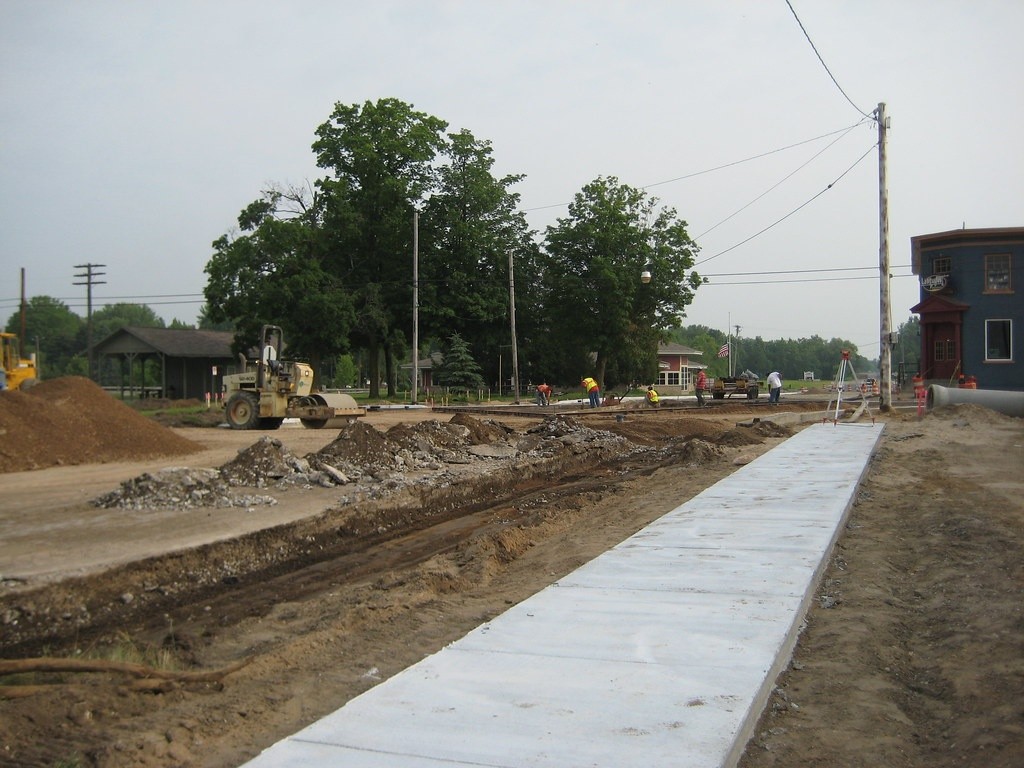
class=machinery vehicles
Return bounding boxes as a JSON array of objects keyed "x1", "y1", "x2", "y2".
[
  {"x1": 0, "y1": 333, "x2": 36, "y2": 392},
  {"x1": 221, "y1": 324, "x2": 368, "y2": 429},
  {"x1": 710, "y1": 377, "x2": 765, "y2": 400}
]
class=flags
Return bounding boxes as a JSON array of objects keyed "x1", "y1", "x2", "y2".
[{"x1": 717, "y1": 344, "x2": 729, "y2": 358}]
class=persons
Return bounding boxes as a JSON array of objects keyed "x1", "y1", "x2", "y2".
[
  {"x1": 695, "y1": 365, "x2": 708, "y2": 408},
  {"x1": 581, "y1": 375, "x2": 601, "y2": 408},
  {"x1": 766, "y1": 370, "x2": 783, "y2": 406},
  {"x1": 645, "y1": 386, "x2": 659, "y2": 408},
  {"x1": 536, "y1": 384, "x2": 553, "y2": 407}
]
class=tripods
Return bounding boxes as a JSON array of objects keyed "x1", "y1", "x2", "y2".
[{"x1": 822, "y1": 358, "x2": 875, "y2": 427}]
